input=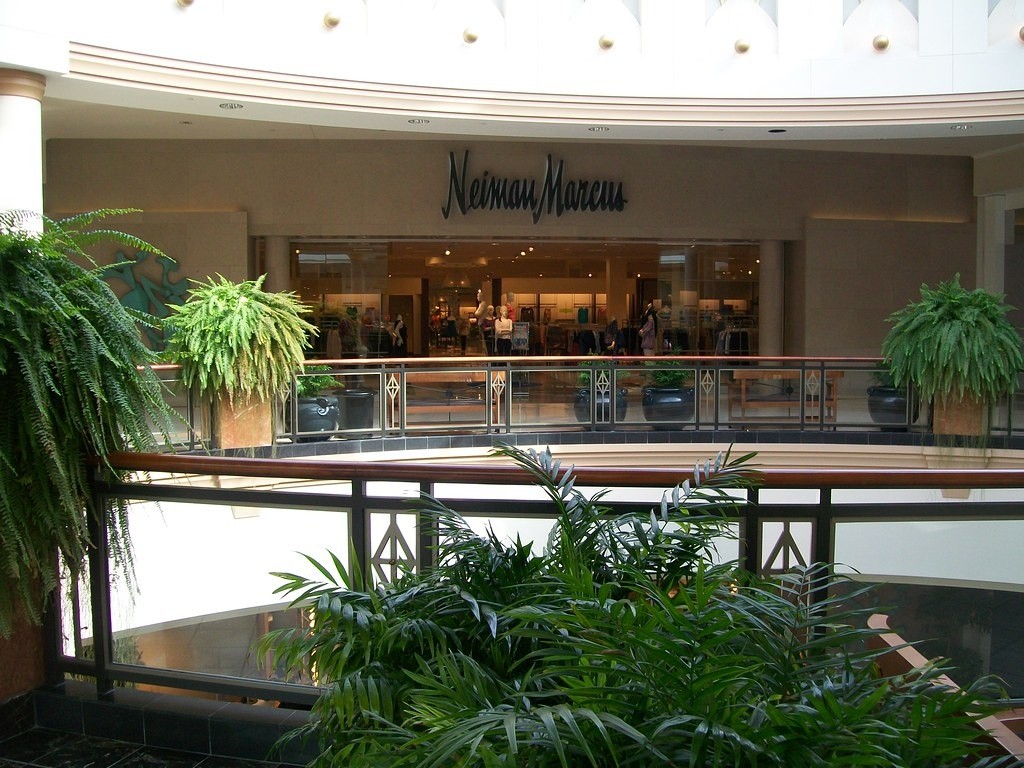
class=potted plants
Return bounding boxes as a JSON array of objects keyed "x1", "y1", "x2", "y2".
[
  {"x1": 866, "y1": 359, "x2": 922, "y2": 432},
  {"x1": 280, "y1": 365, "x2": 346, "y2": 443},
  {"x1": 880, "y1": 272, "x2": 1024, "y2": 436},
  {"x1": 640, "y1": 344, "x2": 695, "y2": 431},
  {"x1": 574, "y1": 352, "x2": 632, "y2": 431},
  {"x1": 157, "y1": 271, "x2": 320, "y2": 449}
]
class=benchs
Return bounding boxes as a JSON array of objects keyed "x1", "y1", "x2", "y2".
[
  {"x1": 388, "y1": 370, "x2": 506, "y2": 436},
  {"x1": 720, "y1": 368, "x2": 845, "y2": 429}
]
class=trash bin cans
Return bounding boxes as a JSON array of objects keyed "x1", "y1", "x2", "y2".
[{"x1": 332, "y1": 388, "x2": 378, "y2": 440}]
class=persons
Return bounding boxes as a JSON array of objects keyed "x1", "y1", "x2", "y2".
[
  {"x1": 394, "y1": 313, "x2": 408, "y2": 365},
  {"x1": 430, "y1": 290, "x2": 512, "y2": 366},
  {"x1": 252, "y1": 678, "x2": 283, "y2": 708},
  {"x1": 583, "y1": 315, "x2": 618, "y2": 356},
  {"x1": 638, "y1": 303, "x2": 657, "y2": 388},
  {"x1": 338, "y1": 305, "x2": 361, "y2": 389}
]
